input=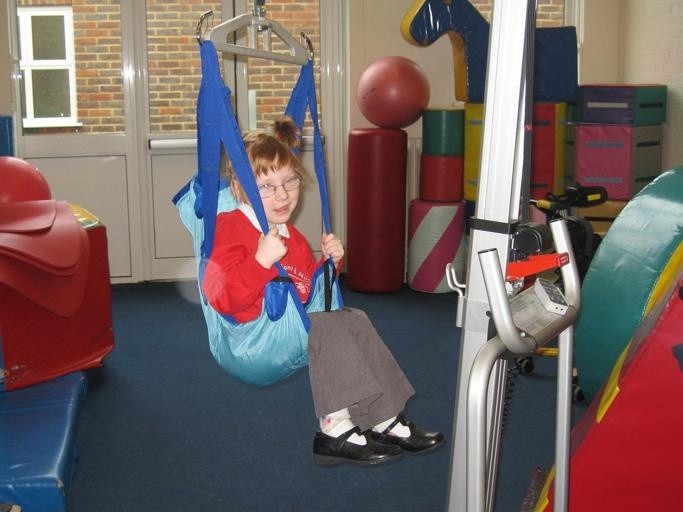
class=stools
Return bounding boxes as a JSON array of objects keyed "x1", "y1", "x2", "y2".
[
  {"x1": 406, "y1": 202, "x2": 468, "y2": 290},
  {"x1": 421, "y1": 107, "x2": 463, "y2": 154},
  {"x1": 416, "y1": 154, "x2": 464, "y2": 201}
]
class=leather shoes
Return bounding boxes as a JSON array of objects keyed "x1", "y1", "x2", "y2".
[
  {"x1": 313, "y1": 425, "x2": 402, "y2": 467},
  {"x1": 370, "y1": 413, "x2": 445, "y2": 456}
]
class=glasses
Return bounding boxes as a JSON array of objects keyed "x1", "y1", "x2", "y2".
[{"x1": 253, "y1": 175, "x2": 301, "y2": 198}]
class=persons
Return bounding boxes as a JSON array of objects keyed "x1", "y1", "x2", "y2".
[{"x1": 199, "y1": 115, "x2": 447, "y2": 467}]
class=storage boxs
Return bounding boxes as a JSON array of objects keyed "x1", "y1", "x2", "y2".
[
  {"x1": 463, "y1": 103, "x2": 567, "y2": 199},
  {"x1": 576, "y1": 80, "x2": 669, "y2": 120},
  {"x1": 572, "y1": 123, "x2": 668, "y2": 202}
]
